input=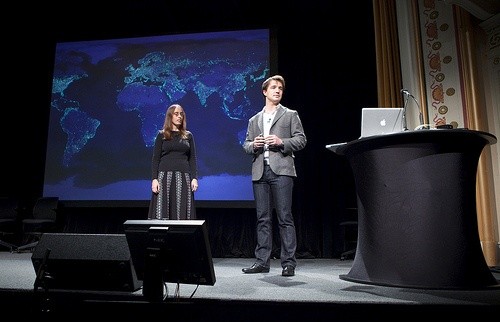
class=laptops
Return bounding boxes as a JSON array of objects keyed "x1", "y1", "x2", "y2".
[{"x1": 361, "y1": 108, "x2": 406, "y2": 138}]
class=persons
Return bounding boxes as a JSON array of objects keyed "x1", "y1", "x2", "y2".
[
  {"x1": 242, "y1": 75, "x2": 307, "y2": 277},
  {"x1": 151, "y1": 104, "x2": 198, "y2": 220}
]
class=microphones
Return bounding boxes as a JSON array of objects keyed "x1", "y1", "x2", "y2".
[{"x1": 400, "y1": 88, "x2": 424, "y2": 131}]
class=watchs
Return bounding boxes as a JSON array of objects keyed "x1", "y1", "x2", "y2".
[{"x1": 280, "y1": 140, "x2": 284, "y2": 149}]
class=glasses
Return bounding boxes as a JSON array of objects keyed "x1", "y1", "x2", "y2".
[{"x1": 172, "y1": 113, "x2": 183, "y2": 115}]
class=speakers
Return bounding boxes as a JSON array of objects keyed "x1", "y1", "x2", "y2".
[{"x1": 31, "y1": 232, "x2": 143, "y2": 292}]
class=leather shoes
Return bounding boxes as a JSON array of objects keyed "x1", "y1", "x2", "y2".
[
  {"x1": 242, "y1": 263, "x2": 270, "y2": 273},
  {"x1": 282, "y1": 265, "x2": 295, "y2": 276}
]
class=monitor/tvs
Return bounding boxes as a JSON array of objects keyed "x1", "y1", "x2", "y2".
[{"x1": 124, "y1": 220, "x2": 216, "y2": 304}]
